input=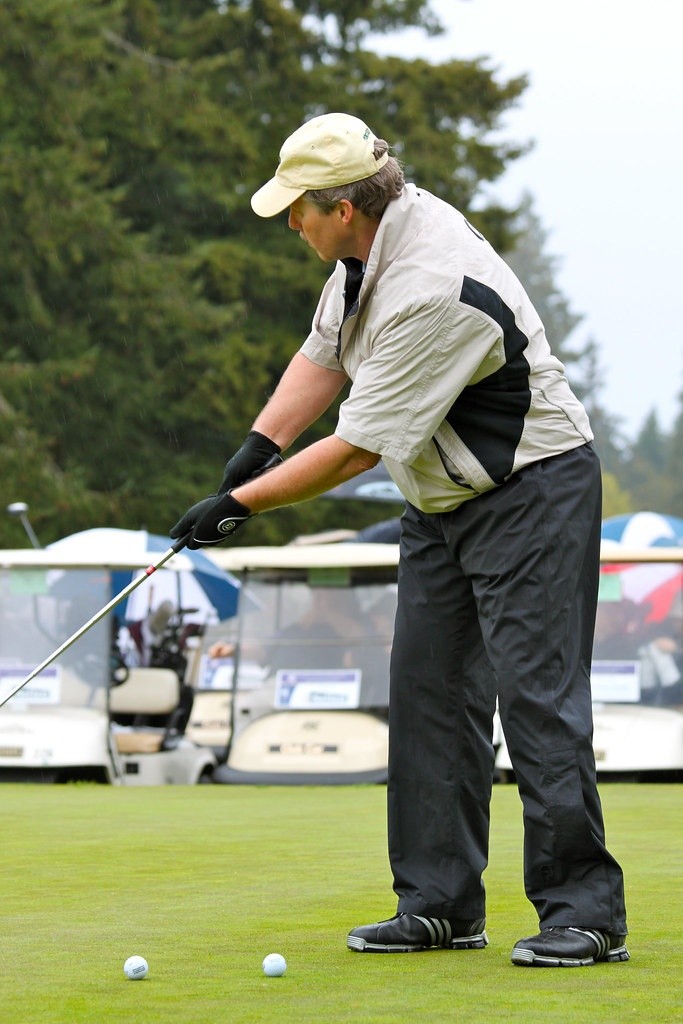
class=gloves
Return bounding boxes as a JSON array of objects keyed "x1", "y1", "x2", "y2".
[
  {"x1": 209, "y1": 430, "x2": 283, "y2": 497},
  {"x1": 169, "y1": 489, "x2": 258, "y2": 551}
]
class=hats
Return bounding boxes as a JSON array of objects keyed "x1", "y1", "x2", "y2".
[{"x1": 251, "y1": 112, "x2": 390, "y2": 218}]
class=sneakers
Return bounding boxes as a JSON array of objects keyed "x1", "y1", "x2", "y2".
[
  {"x1": 345, "y1": 911, "x2": 488, "y2": 952},
  {"x1": 511, "y1": 925, "x2": 630, "y2": 967}
]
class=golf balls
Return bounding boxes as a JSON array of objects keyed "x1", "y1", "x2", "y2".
[
  {"x1": 262, "y1": 953, "x2": 287, "y2": 977},
  {"x1": 124, "y1": 955, "x2": 147, "y2": 979}
]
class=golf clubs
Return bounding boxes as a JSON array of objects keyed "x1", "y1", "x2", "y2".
[{"x1": 0, "y1": 454, "x2": 283, "y2": 709}]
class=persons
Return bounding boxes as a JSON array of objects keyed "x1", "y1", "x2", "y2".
[{"x1": 167, "y1": 110, "x2": 640, "y2": 972}]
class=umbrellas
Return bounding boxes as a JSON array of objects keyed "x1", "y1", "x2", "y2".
[
  {"x1": 30, "y1": 517, "x2": 263, "y2": 646},
  {"x1": 582, "y1": 506, "x2": 683, "y2": 556}
]
